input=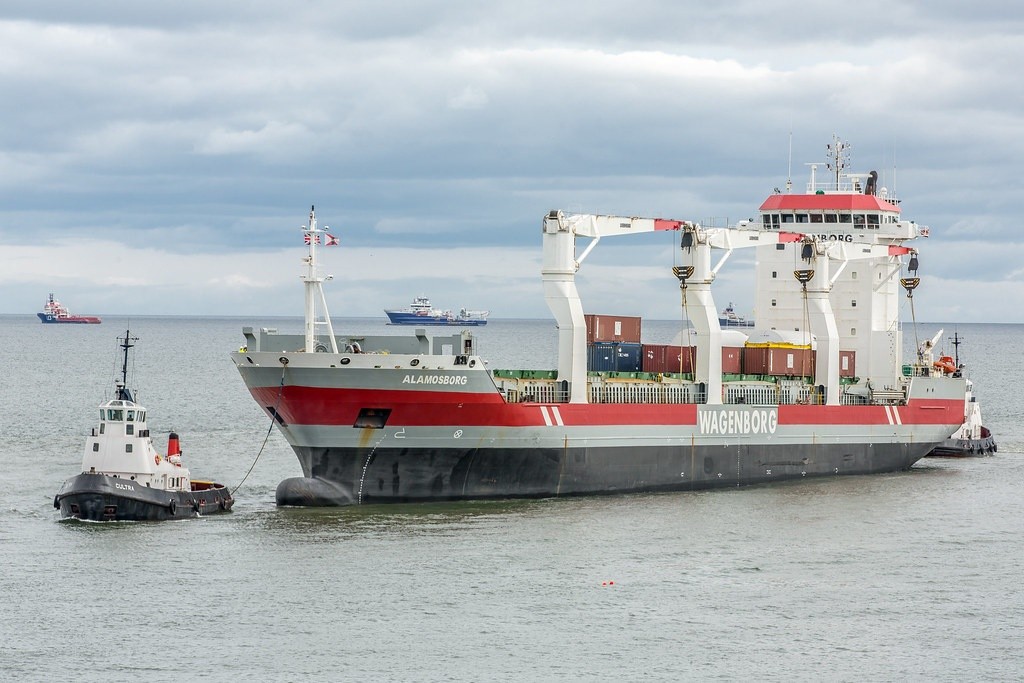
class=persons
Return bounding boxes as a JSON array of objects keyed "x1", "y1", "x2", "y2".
[{"x1": 917, "y1": 350, "x2": 923, "y2": 362}]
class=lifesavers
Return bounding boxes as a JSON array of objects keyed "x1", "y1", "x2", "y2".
[
  {"x1": 54, "y1": 494, "x2": 60, "y2": 509},
  {"x1": 220, "y1": 499, "x2": 225, "y2": 508},
  {"x1": 170, "y1": 499, "x2": 177, "y2": 515},
  {"x1": 195, "y1": 501, "x2": 201, "y2": 512},
  {"x1": 155, "y1": 455, "x2": 159, "y2": 463}
]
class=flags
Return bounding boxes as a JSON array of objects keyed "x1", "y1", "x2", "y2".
[
  {"x1": 305, "y1": 234, "x2": 321, "y2": 246},
  {"x1": 325, "y1": 234, "x2": 339, "y2": 246}
]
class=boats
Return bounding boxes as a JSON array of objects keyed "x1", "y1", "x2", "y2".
[
  {"x1": 37, "y1": 293, "x2": 103, "y2": 324},
  {"x1": 228, "y1": 134, "x2": 969, "y2": 502},
  {"x1": 718, "y1": 302, "x2": 756, "y2": 330},
  {"x1": 381, "y1": 292, "x2": 489, "y2": 327},
  {"x1": 901, "y1": 331, "x2": 999, "y2": 458},
  {"x1": 53, "y1": 327, "x2": 235, "y2": 522}
]
